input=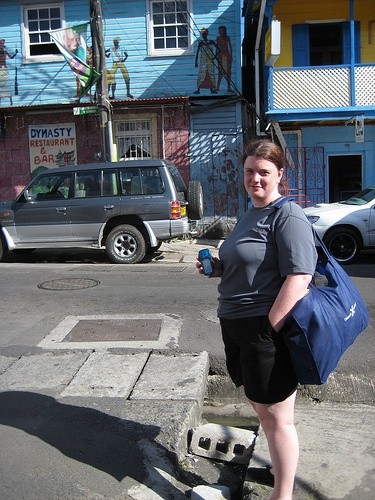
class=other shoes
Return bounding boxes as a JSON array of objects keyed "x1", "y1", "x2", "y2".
[{"x1": 247, "y1": 467, "x2": 295, "y2": 490}]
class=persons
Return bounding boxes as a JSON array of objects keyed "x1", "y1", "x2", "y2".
[{"x1": 196, "y1": 139, "x2": 318, "y2": 500}]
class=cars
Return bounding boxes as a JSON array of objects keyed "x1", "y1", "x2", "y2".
[{"x1": 301, "y1": 183, "x2": 375, "y2": 265}]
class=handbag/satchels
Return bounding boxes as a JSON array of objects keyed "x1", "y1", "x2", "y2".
[{"x1": 268, "y1": 197, "x2": 368, "y2": 385}]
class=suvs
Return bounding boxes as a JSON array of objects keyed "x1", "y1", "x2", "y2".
[{"x1": 0, "y1": 159, "x2": 204, "y2": 264}]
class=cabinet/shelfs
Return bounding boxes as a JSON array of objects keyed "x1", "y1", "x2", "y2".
[{"x1": 339, "y1": 190, "x2": 360, "y2": 201}]
